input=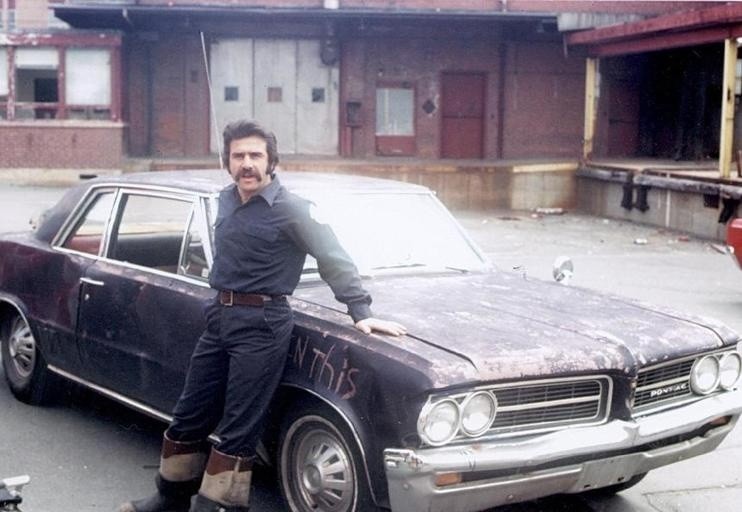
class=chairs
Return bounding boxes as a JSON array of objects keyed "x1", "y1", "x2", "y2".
[{"x1": 114, "y1": 232, "x2": 191, "y2": 267}]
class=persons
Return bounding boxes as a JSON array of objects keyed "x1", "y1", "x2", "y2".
[{"x1": 119, "y1": 117, "x2": 409, "y2": 511}]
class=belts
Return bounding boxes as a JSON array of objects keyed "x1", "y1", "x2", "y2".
[{"x1": 218, "y1": 289, "x2": 272, "y2": 307}]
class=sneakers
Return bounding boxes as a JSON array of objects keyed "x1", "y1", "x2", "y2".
[{"x1": 121, "y1": 491, "x2": 191, "y2": 512}]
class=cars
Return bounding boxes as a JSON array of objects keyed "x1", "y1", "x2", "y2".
[{"x1": 0, "y1": 168, "x2": 742, "y2": 512}]
